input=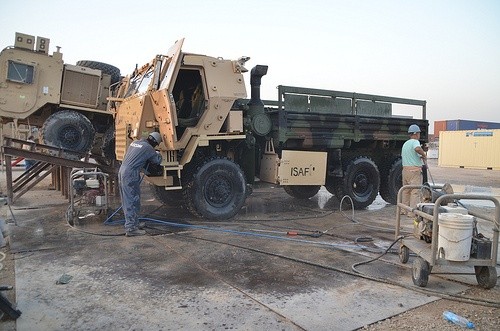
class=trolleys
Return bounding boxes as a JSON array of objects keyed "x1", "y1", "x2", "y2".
[
  {"x1": 395, "y1": 184, "x2": 500, "y2": 289},
  {"x1": 67, "y1": 167, "x2": 113, "y2": 225}
]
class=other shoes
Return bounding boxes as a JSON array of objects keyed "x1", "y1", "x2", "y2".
[
  {"x1": 126, "y1": 229, "x2": 145, "y2": 236},
  {"x1": 138, "y1": 222, "x2": 145, "y2": 229},
  {"x1": 407, "y1": 214, "x2": 414, "y2": 218},
  {"x1": 400, "y1": 211, "x2": 407, "y2": 215}
]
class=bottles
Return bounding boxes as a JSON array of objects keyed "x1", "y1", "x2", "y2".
[{"x1": 443, "y1": 311, "x2": 473, "y2": 329}]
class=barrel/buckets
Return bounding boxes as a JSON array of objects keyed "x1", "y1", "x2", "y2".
[{"x1": 438, "y1": 213, "x2": 474, "y2": 261}]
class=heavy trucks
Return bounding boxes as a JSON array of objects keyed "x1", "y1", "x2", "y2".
[
  {"x1": 106, "y1": 38, "x2": 429, "y2": 223},
  {"x1": 0, "y1": 32, "x2": 126, "y2": 161}
]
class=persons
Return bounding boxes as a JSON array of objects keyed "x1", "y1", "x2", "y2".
[
  {"x1": 400, "y1": 125, "x2": 427, "y2": 217},
  {"x1": 118, "y1": 132, "x2": 163, "y2": 237},
  {"x1": 22, "y1": 135, "x2": 40, "y2": 179}
]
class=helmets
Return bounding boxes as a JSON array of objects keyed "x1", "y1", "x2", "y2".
[
  {"x1": 149, "y1": 131, "x2": 162, "y2": 146},
  {"x1": 408, "y1": 124, "x2": 421, "y2": 133}
]
